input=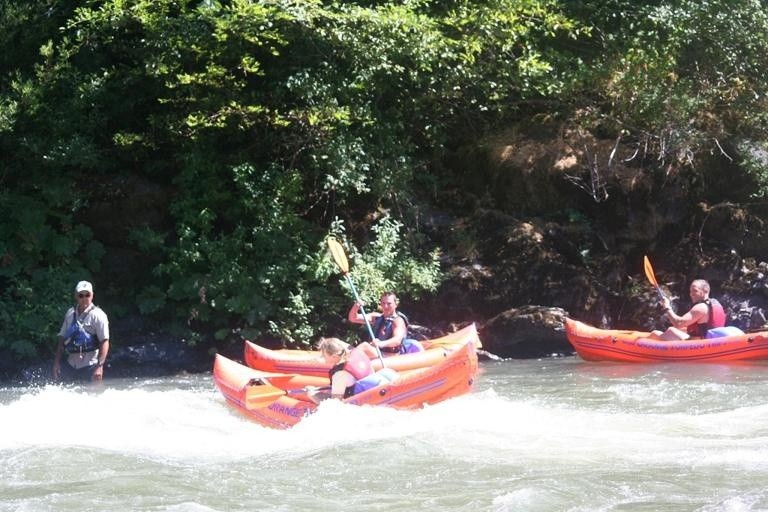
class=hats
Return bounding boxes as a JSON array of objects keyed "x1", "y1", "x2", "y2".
[{"x1": 76, "y1": 281, "x2": 93, "y2": 293}]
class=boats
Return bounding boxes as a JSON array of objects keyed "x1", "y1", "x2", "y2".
[
  {"x1": 559, "y1": 313, "x2": 767, "y2": 366},
  {"x1": 240, "y1": 319, "x2": 485, "y2": 377},
  {"x1": 210, "y1": 333, "x2": 480, "y2": 433}
]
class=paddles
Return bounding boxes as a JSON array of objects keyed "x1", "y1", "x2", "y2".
[
  {"x1": 327, "y1": 240, "x2": 385, "y2": 360},
  {"x1": 642, "y1": 255, "x2": 666, "y2": 302},
  {"x1": 244, "y1": 385, "x2": 333, "y2": 409}
]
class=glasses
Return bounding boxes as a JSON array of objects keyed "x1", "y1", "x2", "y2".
[{"x1": 77, "y1": 294, "x2": 90, "y2": 299}]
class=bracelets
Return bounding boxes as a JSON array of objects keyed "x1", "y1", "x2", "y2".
[
  {"x1": 97, "y1": 360, "x2": 104, "y2": 368},
  {"x1": 663, "y1": 306, "x2": 671, "y2": 314}
]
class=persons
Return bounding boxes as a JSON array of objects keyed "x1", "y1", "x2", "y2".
[
  {"x1": 348, "y1": 292, "x2": 408, "y2": 357},
  {"x1": 53, "y1": 280, "x2": 110, "y2": 386},
  {"x1": 647, "y1": 279, "x2": 725, "y2": 340},
  {"x1": 306, "y1": 337, "x2": 375, "y2": 406}
]
